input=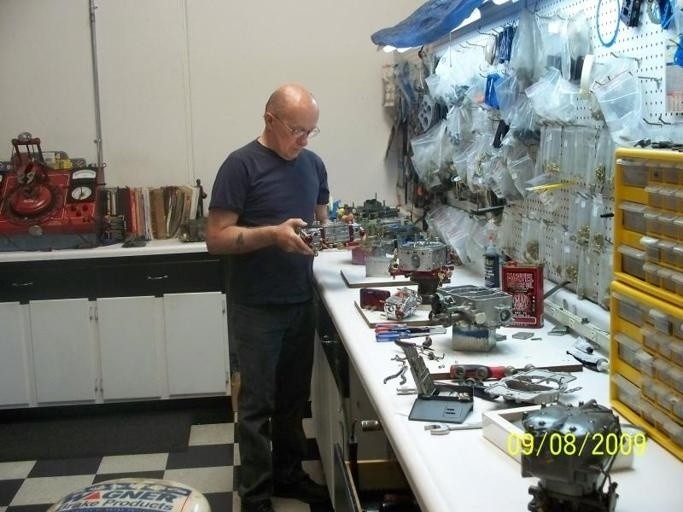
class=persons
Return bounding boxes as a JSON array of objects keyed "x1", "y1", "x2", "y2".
[
  {"x1": 202, "y1": 83, "x2": 367, "y2": 511},
  {"x1": 195, "y1": 179, "x2": 207, "y2": 219}
]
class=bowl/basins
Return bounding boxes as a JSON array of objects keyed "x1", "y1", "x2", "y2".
[{"x1": 350, "y1": 248, "x2": 395, "y2": 278}]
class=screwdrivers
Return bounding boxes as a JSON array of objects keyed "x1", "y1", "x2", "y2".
[{"x1": 375, "y1": 324, "x2": 446, "y2": 341}]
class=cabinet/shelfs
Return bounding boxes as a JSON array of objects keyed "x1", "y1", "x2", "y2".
[
  {"x1": 0, "y1": 253, "x2": 227, "y2": 408},
  {"x1": 310, "y1": 291, "x2": 391, "y2": 512}
]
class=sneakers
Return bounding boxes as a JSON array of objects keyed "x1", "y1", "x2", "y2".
[
  {"x1": 240, "y1": 498, "x2": 275, "y2": 512},
  {"x1": 273, "y1": 473, "x2": 329, "y2": 504}
]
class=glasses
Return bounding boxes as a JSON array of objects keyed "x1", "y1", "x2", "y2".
[{"x1": 274, "y1": 116, "x2": 322, "y2": 138}]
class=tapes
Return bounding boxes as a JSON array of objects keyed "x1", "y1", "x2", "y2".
[{"x1": 580, "y1": 54, "x2": 596, "y2": 99}]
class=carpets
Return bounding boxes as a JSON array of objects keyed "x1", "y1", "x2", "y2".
[{"x1": 0, "y1": 400, "x2": 196, "y2": 462}]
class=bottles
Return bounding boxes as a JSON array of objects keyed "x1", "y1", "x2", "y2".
[{"x1": 484, "y1": 239, "x2": 500, "y2": 288}]
